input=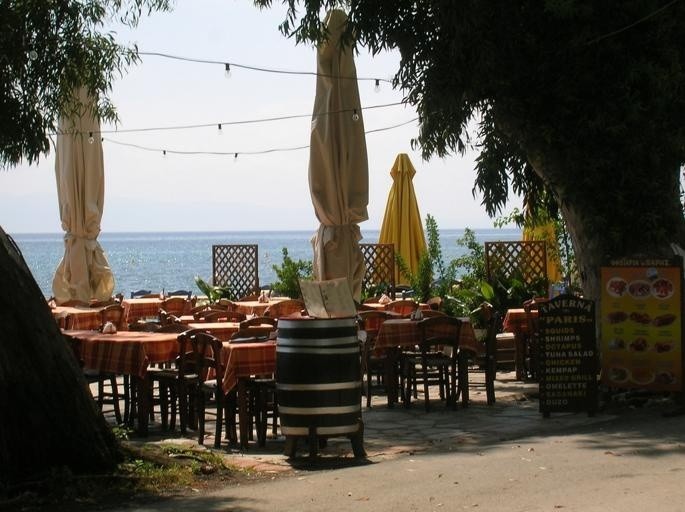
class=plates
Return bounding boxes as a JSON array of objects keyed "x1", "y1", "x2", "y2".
[
  {"x1": 606, "y1": 277, "x2": 674, "y2": 302},
  {"x1": 606, "y1": 365, "x2": 678, "y2": 386}
]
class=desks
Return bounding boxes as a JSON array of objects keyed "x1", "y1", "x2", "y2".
[
  {"x1": 380, "y1": 317, "x2": 474, "y2": 407},
  {"x1": 49, "y1": 306, "x2": 103, "y2": 331},
  {"x1": 123, "y1": 298, "x2": 164, "y2": 327},
  {"x1": 221, "y1": 339, "x2": 277, "y2": 448},
  {"x1": 59, "y1": 329, "x2": 181, "y2": 423},
  {"x1": 229, "y1": 301, "x2": 275, "y2": 319},
  {"x1": 505, "y1": 308, "x2": 539, "y2": 380},
  {"x1": 187, "y1": 321, "x2": 274, "y2": 341}
]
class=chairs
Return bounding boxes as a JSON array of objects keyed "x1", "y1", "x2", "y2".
[
  {"x1": 45, "y1": 288, "x2": 306, "y2": 449},
  {"x1": 351, "y1": 289, "x2": 552, "y2": 408}
]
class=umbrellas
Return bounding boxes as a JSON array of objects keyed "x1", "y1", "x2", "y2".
[
  {"x1": 306, "y1": 8, "x2": 370, "y2": 306},
  {"x1": 374, "y1": 153, "x2": 432, "y2": 291},
  {"x1": 523, "y1": 192, "x2": 562, "y2": 286},
  {"x1": 49, "y1": 39, "x2": 114, "y2": 307}
]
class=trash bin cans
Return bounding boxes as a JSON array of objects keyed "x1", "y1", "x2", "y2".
[{"x1": 275, "y1": 311, "x2": 366, "y2": 458}]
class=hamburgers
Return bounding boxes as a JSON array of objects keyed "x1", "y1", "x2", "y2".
[{"x1": 632, "y1": 369, "x2": 652, "y2": 382}]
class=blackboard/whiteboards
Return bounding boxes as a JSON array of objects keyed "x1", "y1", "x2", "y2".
[{"x1": 539, "y1": 295, "x2": 596, "y2": 414}]
